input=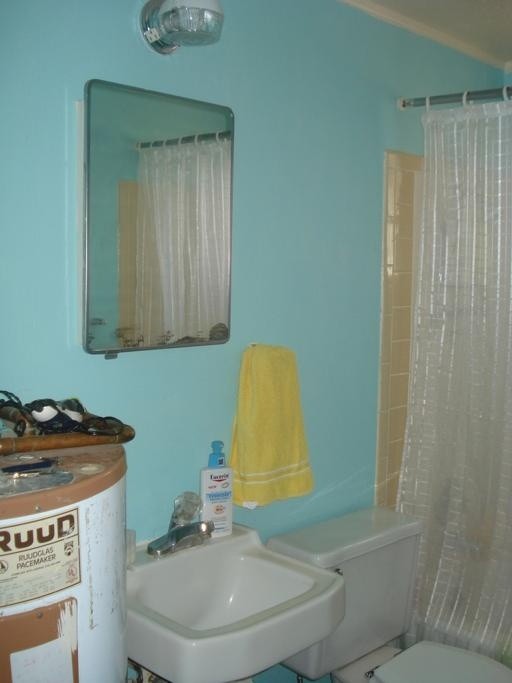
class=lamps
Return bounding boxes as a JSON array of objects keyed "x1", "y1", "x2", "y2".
[{"x1": 139, "y1": 0, "x2": 224, "y2": 54}]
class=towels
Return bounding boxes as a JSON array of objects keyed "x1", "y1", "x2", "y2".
[{"x1": 229, "y1": 346, "x2": 315, "y2": 508}]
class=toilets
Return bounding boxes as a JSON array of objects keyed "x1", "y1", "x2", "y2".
[{"x1": 270, "y1": 509, "x2": 511, "y2": 683}]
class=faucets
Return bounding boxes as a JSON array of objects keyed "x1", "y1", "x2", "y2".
[{"x1": 147, "y1": 492, "x2": 214, "y2": 552}]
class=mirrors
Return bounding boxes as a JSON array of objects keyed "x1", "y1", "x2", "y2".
[{"x1": 82, "y1": 80, "x2": 234, "y2": 354}]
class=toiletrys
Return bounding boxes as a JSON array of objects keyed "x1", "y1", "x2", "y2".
[{"x1": 199, "y1": 441, "x2": 234, "y2": 536}]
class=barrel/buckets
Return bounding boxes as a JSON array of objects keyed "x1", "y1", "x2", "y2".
[{"x1": 1, "y1": 442, "x2": 129, "y2": 683}]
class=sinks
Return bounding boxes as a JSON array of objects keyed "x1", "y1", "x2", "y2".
[{"x1": 121, "y1": 527, "x2": 345, "y2": 683}]
